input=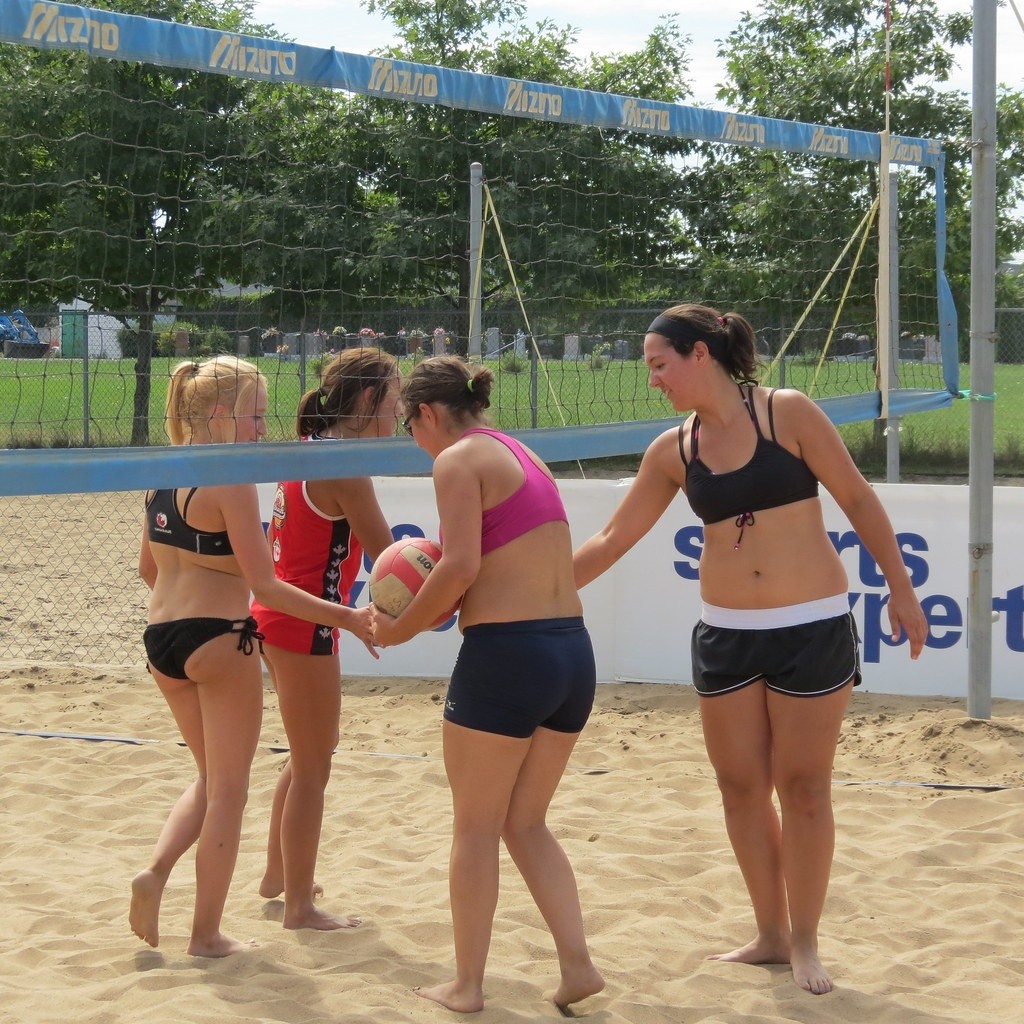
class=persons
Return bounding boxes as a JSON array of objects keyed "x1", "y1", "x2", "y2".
[
  {"x1": 572, "y1": 300, "x2": 930, "y2": 996},
  {"x1": 129, "y1": 348, "x2": 604, "y2": 1013}
]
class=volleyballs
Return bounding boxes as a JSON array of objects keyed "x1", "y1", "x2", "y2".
[{"x1": 367, "y1": 537, "x2": 462, "y2": 633}]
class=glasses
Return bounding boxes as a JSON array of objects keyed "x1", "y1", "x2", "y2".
[{"x1": 402, "y1": 408, "x2": 419, "y2": 437}]
